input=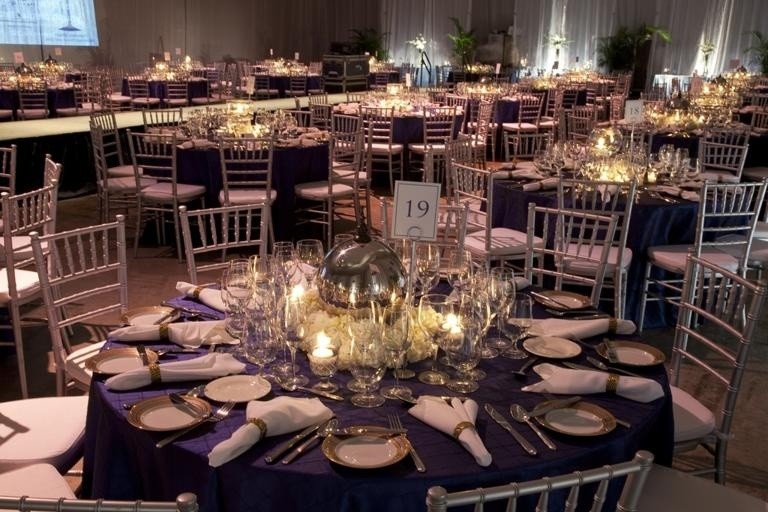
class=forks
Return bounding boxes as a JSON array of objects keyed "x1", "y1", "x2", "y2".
[
  {"x1": 385, "y1": 411, "x2": 428, "y2": 475},
  {"x1": 155, "y1": 398, "x2": 237, "y2": 450},
  {"x1": 288, "y1": 419, "x2": 341, "y2": 466}
]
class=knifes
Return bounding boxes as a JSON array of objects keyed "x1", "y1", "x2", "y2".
[
  {"x1": 166, "y1": 393, "x2": 210, "y2": 418},
  {"x1": 264, "y1": 413, "x2": 336, "y2": 465},
  {"x1": 527, "y1": 289, "x2": 573, "y2": 313},
  {"x1": 526, "y1": 396, "x2": 584, "y2": 419},
  {"x1": 484, "y1": 404, "x2": 538, "y2": 457}
]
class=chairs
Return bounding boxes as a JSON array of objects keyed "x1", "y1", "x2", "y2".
[{"x1": 0, "y1": 53, "x2": 768, "y2": 512}]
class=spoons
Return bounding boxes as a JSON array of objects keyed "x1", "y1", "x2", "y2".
[
  {"x1": 508, "y1": 401, "x2": 559, "y2": 451},
  {"x1": 587, "y1": 357, "x2": 649, "y2": 381}
]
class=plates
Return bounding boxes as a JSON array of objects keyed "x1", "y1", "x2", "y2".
[
  {"x1": 532, "y1": 397, "x2": 620, "y2": 437},
  {"x1": 532, "y1": 288, "x2": 594, "y2": 312},
  {"x1": 119, "y1": 305, "x2": 182, "y2": 326},
  {"x1": 319, "y1": 424, "x2": 411, "y2": 471},
  {"x1": 592, "y1": 340, "x2": 668, "y2": 367},
  {"x1": 202, "y1": 374, "x2": 273, "y2": 406},
  {"x1": 520, "y1": 334, "x2": 584, "y2": 363},
  {"x1": 125, "y1": 392, "x2": 213, "y2": 434},
  {"x1": 84, "y1": 346, "x2": 161, "y2": 377}
]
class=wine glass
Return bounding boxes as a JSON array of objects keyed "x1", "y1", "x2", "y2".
[
  {"x1": 535, "y1": 136, "x2": 692, "y2": 186},
  {"x1": 222, "y1": 230, "x2": 539, "y2": 408}
]
